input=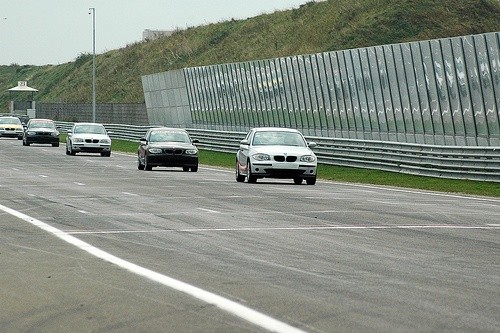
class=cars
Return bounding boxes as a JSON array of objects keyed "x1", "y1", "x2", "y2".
[
  {"x1": 66, "y1": 123, "x2": 111, "y2": 157},
  {"x1": 0, "y1": 116, "x2": 23, "y2": 140},
  {"x1": 138, "y1": 127, "x2": 199, "y2": 172},
  {"x1": 23, "y1": 119, "x2": 61, "y2": 147},
  {"x1": 236, "y1": 127, "x2": 317, "y2": 185}
]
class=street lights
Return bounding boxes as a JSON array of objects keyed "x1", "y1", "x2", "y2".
[{"x1": 89, "y1": 8, "x2": 95, "y2": 125}]
